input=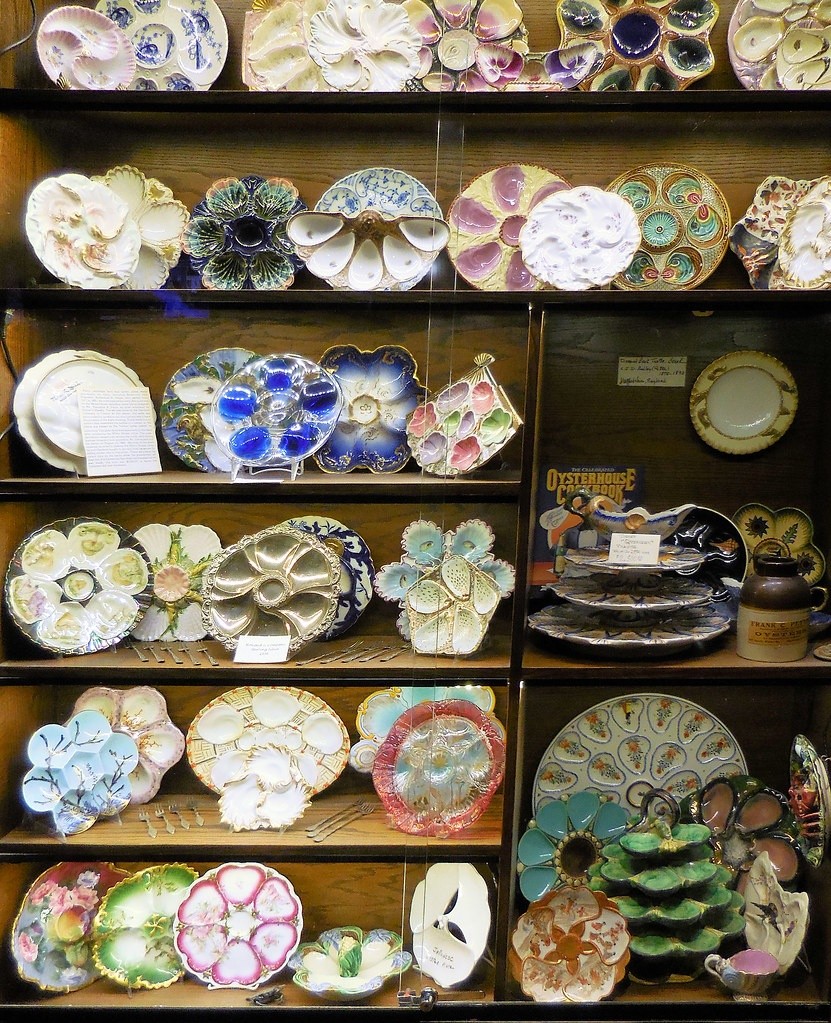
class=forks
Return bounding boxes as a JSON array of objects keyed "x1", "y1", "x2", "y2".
[
  {"x1": 139, "y1": 804, "x2": 159, "y2": 838},
  {"x1": 123, "y1": 636, "x2": 149, "y2": 662},
  {"x1": 177, "y1": 640, "x2": 202, "y2": 665},
  {"x1": 141, "y1": 639, "x2": 165, "y2": 663},
  {"x1": 295, "y1": 640, "x2": 411, "y2": 663},
  {"x1": 154, "y1": 802, "x2": 176, "y2": 834},
  {"x1": 195, "y1": 641, "x2": 220, "y2": 666},
  {"x1": 158, "y1": 640, "x2": 184, "y2": 664},
  {"x1": 168, "y1": 800, "x2": 189, "y2": 830},
  {"x1": 187, "y1": 796, "x2": 205, "y2": 827},
  {"x1": 304, "y1": 794, "x2": 375, "y2": 843}
]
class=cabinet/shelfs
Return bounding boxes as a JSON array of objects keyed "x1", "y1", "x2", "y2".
[{"x1": 0, "y1": 0, "x2": 831, "y2": 1023}]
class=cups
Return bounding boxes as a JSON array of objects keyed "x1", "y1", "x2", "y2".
[
  {"x1": 737, "y1": 555, "x2": 828, "y2": 661},
  {"x1": 704, "y1": 949, "x2": 780, "y2": 1002}
]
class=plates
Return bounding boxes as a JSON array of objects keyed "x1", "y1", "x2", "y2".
[
  {"x1": 508, "y1": 887, "x2": 632, "y2": 1001},
  {"x1": 662, "y1": 506, "x2": 748, "y2": 600},
  {"x1": 409, "y1": 863, "x2": 491, "y2": 988},
  {"x1": 186, "y1": 686, "x2": 351, "y2": 833},
  {"x1": 63, "y1": 686, "x2": 185, "y2": 804},
  {"x1": 518, "y1": 692, "x2": 801, "y2": 913},
  {"x1": 200, "y1": 515, "x2": 374, "y2": 656},
  {"x1": 25, "y1": 161, "x2": 828, "y2": 290},
  {"x1": 525, "y1": 602, "x2": 730, "y2": 647},
  {"x1": 160, "y1": 345, "x2": 523, "y2": 479},
  {"x1": 10, "y1": 862, "x2": 201, "y2": 992},
  {"x1": 5, "y1": 517, "x2": 154, "y2": 654},
  {"x1": 132, "y1": 523, "x2": 224, "y2": 642},
  {"x1": 689, "y1": 351, "x2": 798, "y2": 454},
  {"x1": 565, "y1": 545, "x2": 706, "y2": 572},
  {"x1": 36, "y1": 0, "x2": 831, "y2": 91},
  {"x1": 373, "y1": 519, "x2": 515, "y2": 656},
  {"x1": 13, "y1": 351, "x2": 156, "y2": 475},
  {"x1": 349, "y1": 687, "x2": 506, "y2": 837},
  {"x1": 173, "y1": 862, "x2": 304, "y2": 991},
  {"x1": 730, "y1": 503, "x2": 826, "y2": 585},
  {"x1": 543, "y1": 577, "x2": 715, "y2": 616},
  {"x1": 22, "y1": 710, "x2": 139, "y2": 834}
]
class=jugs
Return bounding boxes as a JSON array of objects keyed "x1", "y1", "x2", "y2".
[{"x1": 563, "y1": 488, "x2": 697, "y2": 542}]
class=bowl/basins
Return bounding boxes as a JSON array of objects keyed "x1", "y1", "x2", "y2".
[{"x1": 287, "y1": 925, "x2": 412, "y2": 1002}]
